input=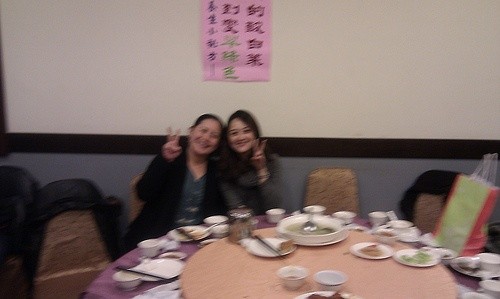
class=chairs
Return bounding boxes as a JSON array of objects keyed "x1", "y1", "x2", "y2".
[
  {"x1": 0, "y1": 164, "x2": 148, "y2": 299},
  {"x1": 402, "y1": 168, "x2": 467, "y2": 234},
  {"x1": 305, "y1": 167, "x2": 360, "y2": 218}
]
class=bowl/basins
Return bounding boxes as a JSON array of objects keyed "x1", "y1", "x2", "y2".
[
  {"x1": 368, "y1": 211, "x2": 387, "y2": 226},
  {"x1": 332, "y1": 211, "x2": 356, "y2": 224},
  {"x1": 112, "y1": 269, "x2": 142, "y2": 292},
  {"x1": 265, "y1": 208, "x2": 286, "y2": 223},
  {"x1": 203, "y1": 215, "x2": 228, "y2": 237},
  {"x1": 138, "y1": 239, "x2": 162, "y2": 258},
  {"x1": 480, "y1": 253, "x2": 500, "y2": 272},
  {"x1": 477, "y1": 279, "x2": 500, "y2": 294},
  {"x1": 388, "y1": 219, "x2": 414, "y2": 233},
  {"x1": 278, "y1": 265, "x2": 310, "y2": 289},
  {"x1": 303, "y1": 205, "x2": 326, "y2": 215},
  {"x1": 313, "y1": 270, "x2": 348, "y2": 291},
  {"x1": 276, "y1": 213, "x2": 346, "y2": 243}
]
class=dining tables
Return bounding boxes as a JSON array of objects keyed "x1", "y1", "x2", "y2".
[{"x1": 83, "y1": 210, "x2": 500, "y2": 299}]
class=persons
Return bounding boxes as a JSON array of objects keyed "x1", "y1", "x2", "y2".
[
  {"x1": 218, "y1": 109, "x2": 281, "y2": 217},
  {"x1": 125, "y1": 114, "x2": 222, "y2": 256}
]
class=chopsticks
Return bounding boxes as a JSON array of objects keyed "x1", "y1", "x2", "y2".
[
  {"x1": 115, "y1": 267, "x2": 167, "y2": 280},
  {"x1": 251, "y1": 235, "x2": 287, "y2": 260}
]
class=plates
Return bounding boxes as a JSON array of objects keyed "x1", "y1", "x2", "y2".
[
  {"x1": 167, "y1": 225, "x2": 210, "y2": 242},
  {"x1": 131, "y1": 258, "x2": 185, "y2": 282},
  {"x1": 349, "y1": 241, "x2": 395, "y2": 260},
  {"x1": 393, "y1": 249, "x2": 441, "y2": 268},
  {"x1": 420, "y1": 247, "x2": 458, "y2": 260},
  {"x1": 158, "y1": 251, "x2": 188, "y2": 260},
  {"x1": 448, "y1": 256, "x2": 500, "y2": 278},
  {"x1": 276, "y1": 232, "x2": 349, "y2": 246},
  {"x1": 246, "y1": 237, "x2": 297, "y2": 257},
  {"x1": 459, "y1": 292, "x2": 494, "y2": 299},
  {"x1": 295, "y1": 291, "x2": 358, "y2": 299}
]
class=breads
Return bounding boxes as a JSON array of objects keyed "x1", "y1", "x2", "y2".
[
  {"x1": 306, "y1": 293, "x2": 345, "y2": 299},
  {"x1": 281, "y1": 240, "x2": 294, "y2": 254}
]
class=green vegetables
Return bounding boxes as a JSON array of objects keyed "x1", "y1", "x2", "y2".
[{"x1": 401, "y1": 252, "x2": 430, "y2": 264}]
